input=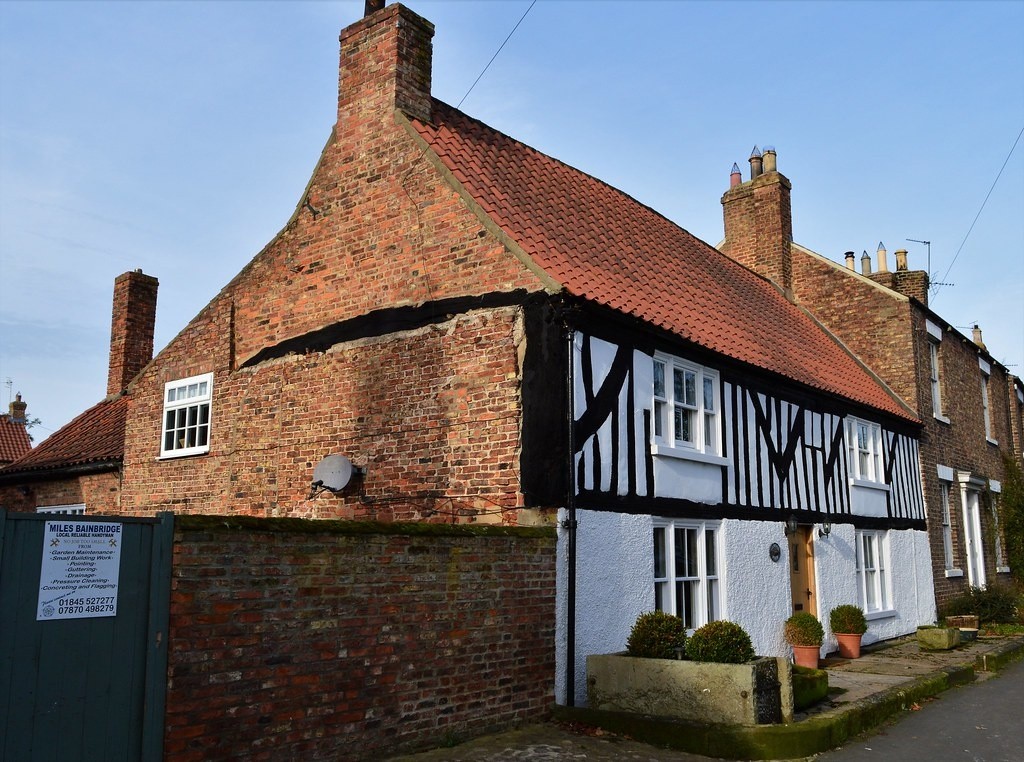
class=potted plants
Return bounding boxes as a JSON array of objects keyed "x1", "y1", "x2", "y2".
[
  {"x1": 945, "y1": 595, "x2": 980, "y2": 629},
  {"x1": 784, "y1": 612, "x2": 826, "y2": 668},
  {"x1": 585, "y1": 610, "x2": 791, "y2": 722},
  {"x1": 829, "y1": 605, "x2": 869, "y2": 659}
]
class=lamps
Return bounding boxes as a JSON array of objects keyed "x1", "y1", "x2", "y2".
[
  {"x1": 785, "y1": 514, "x2": 798, "y2": 536},
  {"x1": 819, "y1": 518, "x2": 831, "y2": 539}
]
